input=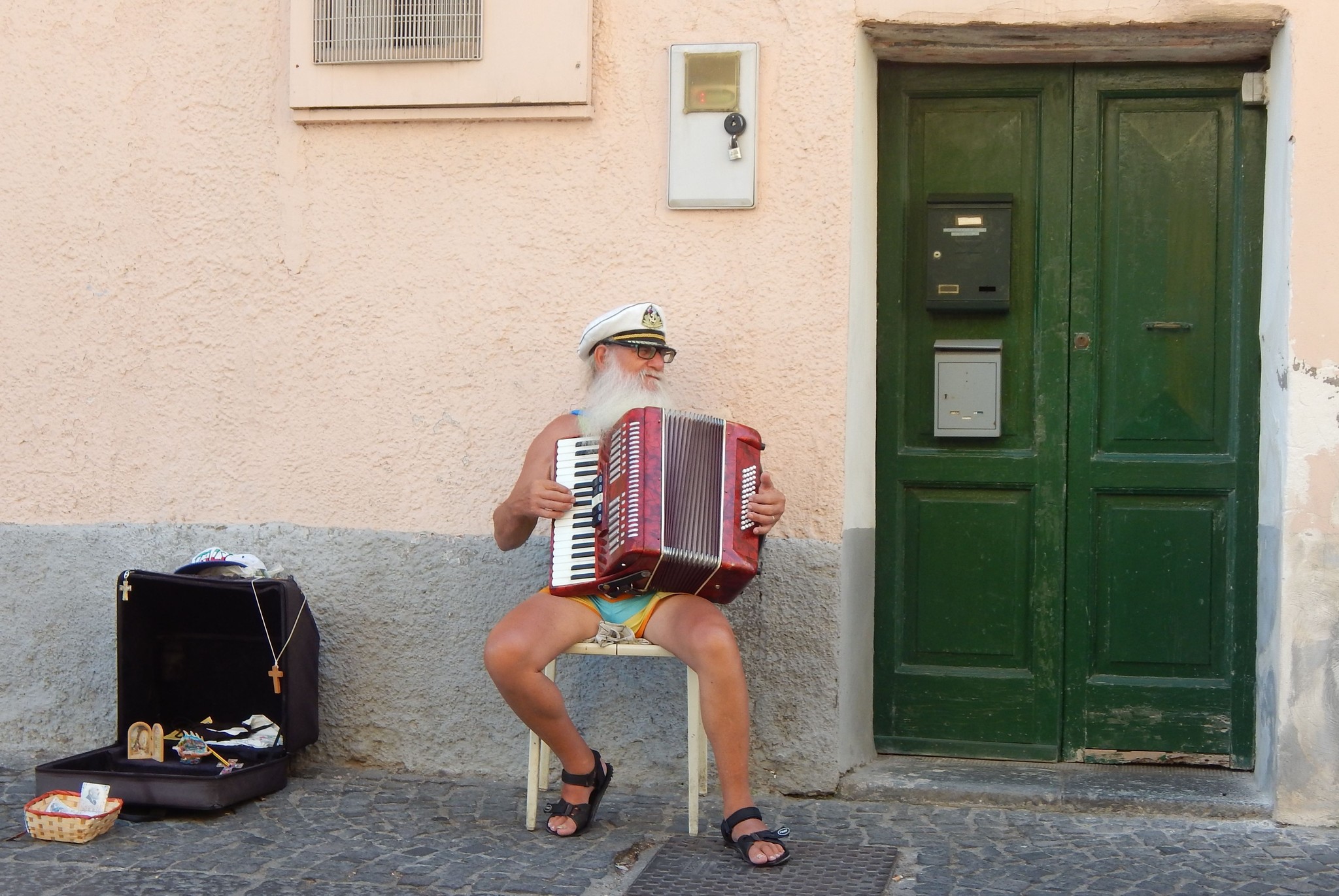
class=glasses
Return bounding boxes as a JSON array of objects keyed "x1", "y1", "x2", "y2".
[{"x1": 608, "y1": 341, "x2": 676, "y2": 363}]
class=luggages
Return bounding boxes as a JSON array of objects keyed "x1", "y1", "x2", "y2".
[{"x1": 35, "y1": 569, "x2": 320, "y2": 822}]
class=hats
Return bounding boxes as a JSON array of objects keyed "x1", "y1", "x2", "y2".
[
  {"x1": 174, "y1": 546, "x2": 272, "y2": 578},
  {"x1": 577, "y1": 301, "x2": 676, "y2": 363}
]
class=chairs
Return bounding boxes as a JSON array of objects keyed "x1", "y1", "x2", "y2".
[{"x1": 526, "y1": 643, "x2": 708, "y2": 836}]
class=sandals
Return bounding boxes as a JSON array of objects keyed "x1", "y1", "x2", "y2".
[
  {"x1": 544, "y1": 748, "x2": 614, "y2": 838},
  {"x1": 720, "y1": 807, "x2": 791, "y2": 866}
]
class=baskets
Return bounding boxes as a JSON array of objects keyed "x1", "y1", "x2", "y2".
[{"x1": 24, "y1": 790, "x2": 123, "y2": 844}]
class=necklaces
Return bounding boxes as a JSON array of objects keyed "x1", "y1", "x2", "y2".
[{"x1": 252, "y1": 575, "x2": 306, "y2": 694}]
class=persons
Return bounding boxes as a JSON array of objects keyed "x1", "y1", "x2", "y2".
[{"x1": 486, "y1": 301, "x2": 794, "y2": 869}]
